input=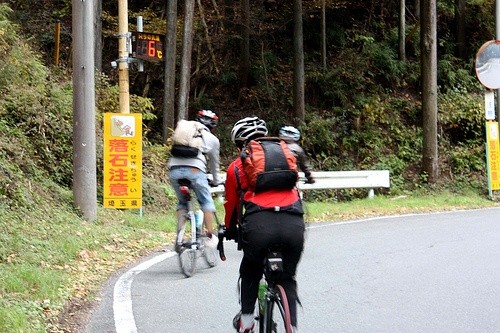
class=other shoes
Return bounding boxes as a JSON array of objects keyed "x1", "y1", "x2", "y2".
[
  {"x1": 206, "y1": 233, "x2": 218, "y2": 247},
  {"x1": 232, "y1": 312, "x2": 257, "y2": 333}
]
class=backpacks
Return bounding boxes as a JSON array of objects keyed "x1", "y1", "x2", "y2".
[
  {"x1": 172, "y1": 119, "x2": 211, "y2": 165},
  {"x1": 240, "y1": 136, "x2": 300, "y2": 195}
]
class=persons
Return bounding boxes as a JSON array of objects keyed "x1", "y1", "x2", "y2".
[
  {"x1": 167, "y1": 109, "x2": 222, "y2": 240},
  {"x1": 224, "y1": 117, "x2": 306, "y2": 333},
  {"x1": 278, "y1": 126, "x2": 315, "y2": 185}
]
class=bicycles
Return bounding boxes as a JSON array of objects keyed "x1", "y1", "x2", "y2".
[
  {"x1": 175, "y1": 179, "x2": 222, "y2": 278},
  {"x1": 218, "y1": 224, "x2": 291, "y2": 333}
]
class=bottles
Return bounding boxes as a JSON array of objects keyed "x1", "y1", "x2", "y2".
[
  {"x1": 258, "y1": 279, "x2": 267, "y2": 309},
  {"x1": 195, "y1": 208, "x2": 204, "y2": 226}
]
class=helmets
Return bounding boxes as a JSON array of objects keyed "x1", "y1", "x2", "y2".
[
  {"x1": 230, "y1": 115, "x2": 269, "y2": 145},
  {"x1": 279, "y1": 126, "x2": 301, "y2": 141},
  {"x1": 198, "y1": 109, "x2": 219, "y2": 127}
]
